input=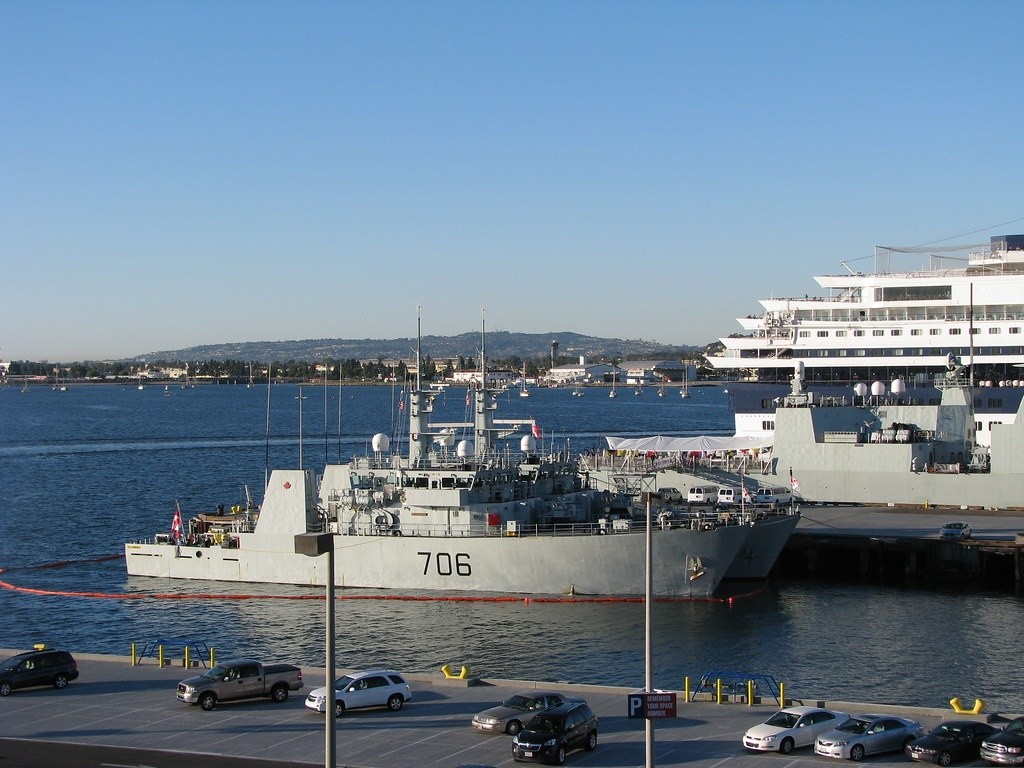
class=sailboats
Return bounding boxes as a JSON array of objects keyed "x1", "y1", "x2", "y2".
[{"x1": 19, "y1": 357, "x2": 697, "y2": 399}]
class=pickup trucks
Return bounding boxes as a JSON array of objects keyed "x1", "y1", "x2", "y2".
[
  {"x1": 656, "y1": 487, "x2": 683, "y2": 504},
  {"x1": 176, "y1": 659, "x2": 306, "y2": 711}
]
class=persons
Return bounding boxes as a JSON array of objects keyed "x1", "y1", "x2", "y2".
[
  {"x1": 591, "y1": 445, "x2": 772, "y2": 467},
  {"x1": 164, "y1": 502, "x2": 262, "y2": 548},
  {"x1": 728, "y1": 294, "x2": 824, "y2": 339},
  {"x1": 996, "y1": 244, "x2": 1023, "y2": 251},
  {"x1": 712, "y1": 501, "x2": 728, "y2": 513}
]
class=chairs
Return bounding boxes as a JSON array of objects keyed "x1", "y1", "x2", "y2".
[
  {"x1": 230, "y1": 668, "x2": 241, "y2": 678},
  {"x1": 359, "y1": 680, "x2": 368, "y2": 689},
  {"x1": 526, "y1": 700, "x2": 543, "y2": 709},
  {"x1": 26, "y1": 661, "x2": 34, "y2": 669},
  {"x1": 874, "y1": 723, "x2": 884, "y2": 732}
]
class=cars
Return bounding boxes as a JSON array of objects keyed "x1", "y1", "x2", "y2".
[
  {"x1": 742, "y1": 706, "x2": 850, "y2": 755},
  {"x1": 471, "y1": 691, "x2": 588, "y2": 735},
  {"x1": 813, "y1": 714, "x2": 924, "y2": 762},
  {"x1": 938, "y1": 521, "x2": 972, "y2": 541},
  {"x1": 905, "y1": 721, "x2": 1000, "y2": 767},
  {"x1": 980, "y1": 716, "x2": 1024, "y2": 766},
  {"x1": 0, "y1": 648, "x2": 79, "y2": 697}
]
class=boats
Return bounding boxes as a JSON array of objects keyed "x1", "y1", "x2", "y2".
[
  {"x1": 125, "y1": 303, "x2": 802, "y2": 606},
  {"x1": 704, "y1": 223, "x2": 1023, "y2": 388},
  {"x1": 579, "y1": 351, "x2": 1024, "y2": 541}
]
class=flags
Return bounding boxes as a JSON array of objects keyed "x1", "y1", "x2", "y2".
[
  {"x1": 532, "y1": 423, "x2": 540, "y2": 438},
  {"x1": 792, "y1": 477, "x2": 800, "y2": 491},
  {"x1": 466, "y1": 389, "x2": 471, "y2": 407},
  {"x1": 171, "y1": 510, "x2": 181, "y2": 539},
  {"x1": 398, "y1": 400, "x2": 403, "y2": 410},
  {"x1": 743, "y1": 488, "x2": 752, "y2": 504}
]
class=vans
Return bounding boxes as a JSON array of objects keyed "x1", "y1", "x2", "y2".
[
  {"x1": 757, "y1": 486, "x2": 794, "y2": 503},
  {"x1": 687, "y1": 484, "x2": 721, "y2": 506},
  {"x1": 716, "y1": 488, "x2": 746, "y2": 505}
]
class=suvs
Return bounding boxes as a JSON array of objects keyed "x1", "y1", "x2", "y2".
[
  {"x1": 512, "y1": 700, "x2": 598, "y2": 767},
  {"x1": 304, "y1": 670, "x2": 413, "y2": 718}
]
